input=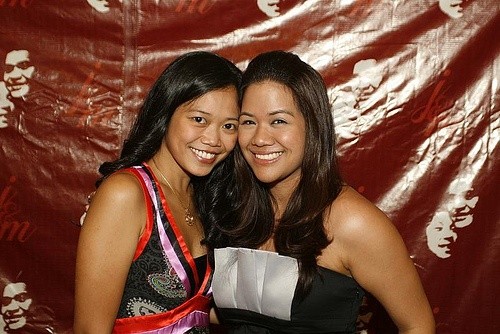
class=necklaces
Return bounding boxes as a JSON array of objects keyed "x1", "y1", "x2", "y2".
[{"x1": 151, "y1": 155, "x2": 197, "y2": 226}]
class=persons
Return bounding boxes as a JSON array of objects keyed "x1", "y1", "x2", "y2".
[
  {"x1": 425, "y1": 175, "x2": 483, "y2": 260},
  {"x1": 73, "y1": 51, "x2": 243, "y2": 334},
  {"x1": 1, "y1": 274, "x2": 57, "y2": 334},
  {"x1": 257, "y1": 0, "x2": 286, "y2": 18},
  {"x1": 356, "y1": 294, "x2": 378, "y2": 334},
  {"x1": 88, "y1": 0, "x2": 121, "y2": 13},
  {"x1": 438, "y1": 0, "x2": 465, "y2": 20},
  {"x1": 0, "y1": 48, "x2": 36, "y2": 129},
  {"x1": 79, "y1": 50, "x2": 436, "y2": 334},
  {"x1": 329, "y1": 85, "x2": 361, "y2": 140}
]
